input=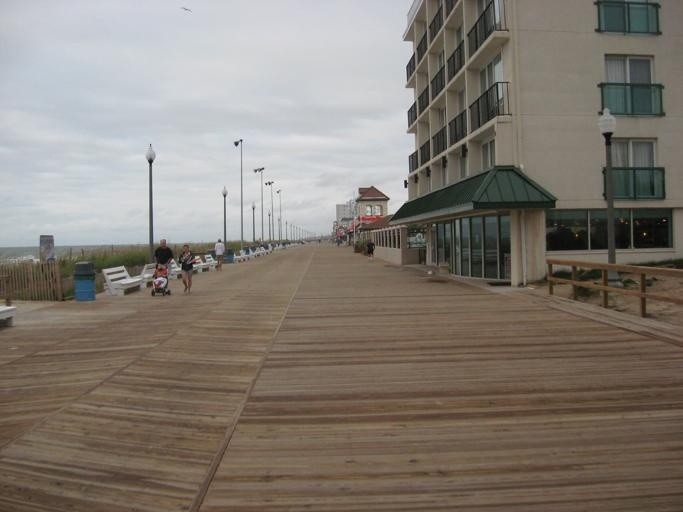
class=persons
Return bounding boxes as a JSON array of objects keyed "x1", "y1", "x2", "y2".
[
  {"x1": 154, "y1": 238, "x2": 173, "y2": 268},
  {"x1": 367, "y1": 238, "x2": 375, "y2": 259},
  {"x1": 158, "y1": 271, "x2": 164, "y2": 277},
  {"x1": 179, "y1": 245, "x2": 196, "y2": 294},
  {"x1": 215, "y1": 238, "x2": 226, "y2": 271}
]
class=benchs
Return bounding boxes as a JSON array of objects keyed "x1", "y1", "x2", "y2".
[
  {"x1": 0, "y1": 303, "x2": 17, "y2": 329},
  {"x1": 100, "y1": 242, "x2": 301, "y2": 297}
]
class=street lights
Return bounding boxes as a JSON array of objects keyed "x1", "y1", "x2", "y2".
[
  {"x1": 220, "y1": 187, "x2": 227, "y2": 250},
  {"x1": 144, "y1": 142, "x2": 156, "y2": 262},
  {"x1": 251, "y1": 167, "x2": 317, "y2": 248},
  {"x1": 233, "y1": 138, "x2": 244, "y2": 250},
  {"x1": 597, "y1": 108, "x2": 621, "y2": 287}
]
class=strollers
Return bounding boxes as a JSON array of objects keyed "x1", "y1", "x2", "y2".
[{"x1": 150, "y1": 262, "x2": 173, "y2": 297}]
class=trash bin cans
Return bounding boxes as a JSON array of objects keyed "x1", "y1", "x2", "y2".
[
  {"x1": 250, "y1": 245, "x2": 256, "y2": 253},
  {"x1": 243, "y1": 246, "x2": 249, "y2": 255},
  {"x1": 72, "y1": 261, "x2": 96, "y2": 301},
  {"x1": 224, "y1": 248, "x2": 234, "y2": 262},
  {"x1": 208, "y1": 249, "x2": 215, "y2": 260}
]
